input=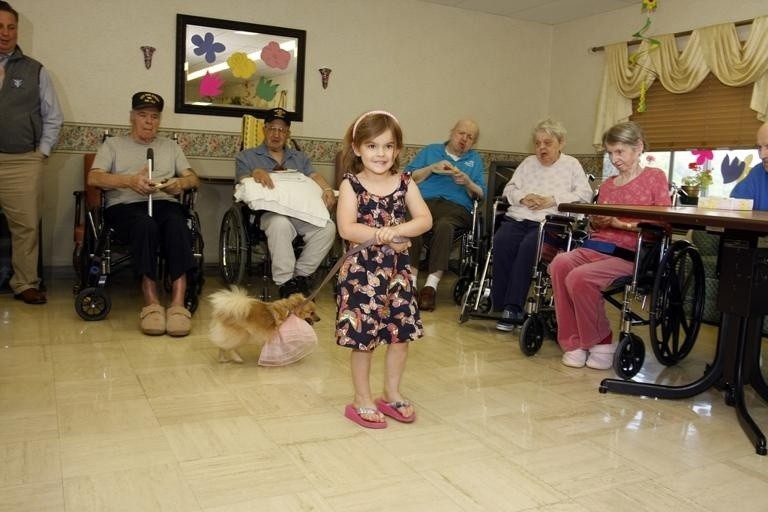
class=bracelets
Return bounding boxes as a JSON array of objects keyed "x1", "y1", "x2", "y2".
[
  {"x1": 625, "y1": 222, "x2": 632, "y2": 231},
  {"x1": 323, "y1": 187, "x2": 333, "y2": 191}
]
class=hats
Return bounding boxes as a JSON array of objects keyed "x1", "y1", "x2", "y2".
[
  {"x1": 132, "y1": 92, "x2": 164, "y2": 112},
  {"x1": 264, "y1": 108, "x2": 290, "y2": 127}
]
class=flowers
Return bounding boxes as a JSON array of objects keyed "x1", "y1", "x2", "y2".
[{"x1": 682, "y1": 162, "x2": 713, "y2": 186}]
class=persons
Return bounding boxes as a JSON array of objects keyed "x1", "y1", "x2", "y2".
[
  {"x1": 490, "y1": 118, "x2": 593, "y2": 331},
  {"x1": 235, "y1": 108, "x2": 336, "y2": 304},
  {"x1": 550, "y1": 121, "x2": 673, "y2": 371},
  {"x1": 0, "y1": 0, "x2": 64, "y2": 305},
  {"x1": 704, "y1": 121, "x2": 768, "y2": 385},
  {"x1": 402, "y1": 119, "x2": 487, "y2": 312},
  {"x1": 87, "y1": 90, "x2": 201, "y2": 337},
  {"x1": 337, "y1": 113, "x2": 433, "y2": 430}
]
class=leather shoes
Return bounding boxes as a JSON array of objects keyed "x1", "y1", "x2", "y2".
[
  {"x1": 412, "y1": 286, "x2": 421, "y2": 308},
  {"x1": 14, "y1": 288, "x2": 46, "y2": 304},
  {"x1": 418, "y1": 286, "x2": 436, "y2": 311}
]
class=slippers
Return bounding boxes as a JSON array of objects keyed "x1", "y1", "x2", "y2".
[
  {"x1": 140, "y1": 303, "x2": 166, "y2": 335},
  {"x1": 561, "y1": 348, "x2": 587, "y2": 368},
  {"x1": 165, "y1": 306, "x2": 192, "y2": 336},
  {"x1": 345, "y1": 404, "x2": 387, "y2": 429},
  {"x1": 374, "y1": 397, "x2": 416, "y2": 423},
  {"x1": 585, "y1": 342, "x2": 619, "y2": 370}
]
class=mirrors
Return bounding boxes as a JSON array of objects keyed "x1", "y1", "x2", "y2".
[{"x1": 175, "y1": 14, "x2": 306, "y2": 121}]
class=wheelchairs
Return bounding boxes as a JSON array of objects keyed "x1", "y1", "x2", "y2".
[
  {"x1": 458, "y1": 174, "x2": 598, "y2": 334},
  {"x1": 73, "y1": 132, "x2": 205, "y2": 322},
  {"x1": 218, "y1": 138, "x2": 341, "y2": 305},
  {"x1": 416, "y1": 193, "x2": 485, "y2": 308},
  {"x1": 518, "y1": 180, "x2": 706, "y2": 380}
]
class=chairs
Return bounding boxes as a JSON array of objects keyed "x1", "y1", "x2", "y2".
[{"x1": 681, "y1": 226, "x2": 767, "y2": 338}]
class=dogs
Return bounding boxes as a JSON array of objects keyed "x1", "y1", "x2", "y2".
[{"x1": 206, "y1": 284, "x2": 321, "y2": 363}]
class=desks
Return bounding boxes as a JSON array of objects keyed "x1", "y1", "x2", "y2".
[{"x1": 558, "y1": 203, "x2": 768, "y2": 455}]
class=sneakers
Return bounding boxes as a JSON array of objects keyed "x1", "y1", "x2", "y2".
[
  {"x1": 295, "y1": 276, "x2": 314, "y2": 303},
  {"x1": 496, "y1": 309, "x2": 517, "y2": 331},
  {"x1": 279, "y1": 278, "x2": 303, "y2": 298},
  {"x1": 515, "y1": 309, "x2": 529, "y2": 328}
]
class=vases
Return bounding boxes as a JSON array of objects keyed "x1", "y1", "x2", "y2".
[{"x1": 679, "y1": 186, "x2": 701, "y2": 205}]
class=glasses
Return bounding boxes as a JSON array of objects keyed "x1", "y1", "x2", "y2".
[{"x1": 264, "y1": 125, "x2": 286, "y2": 133}]
